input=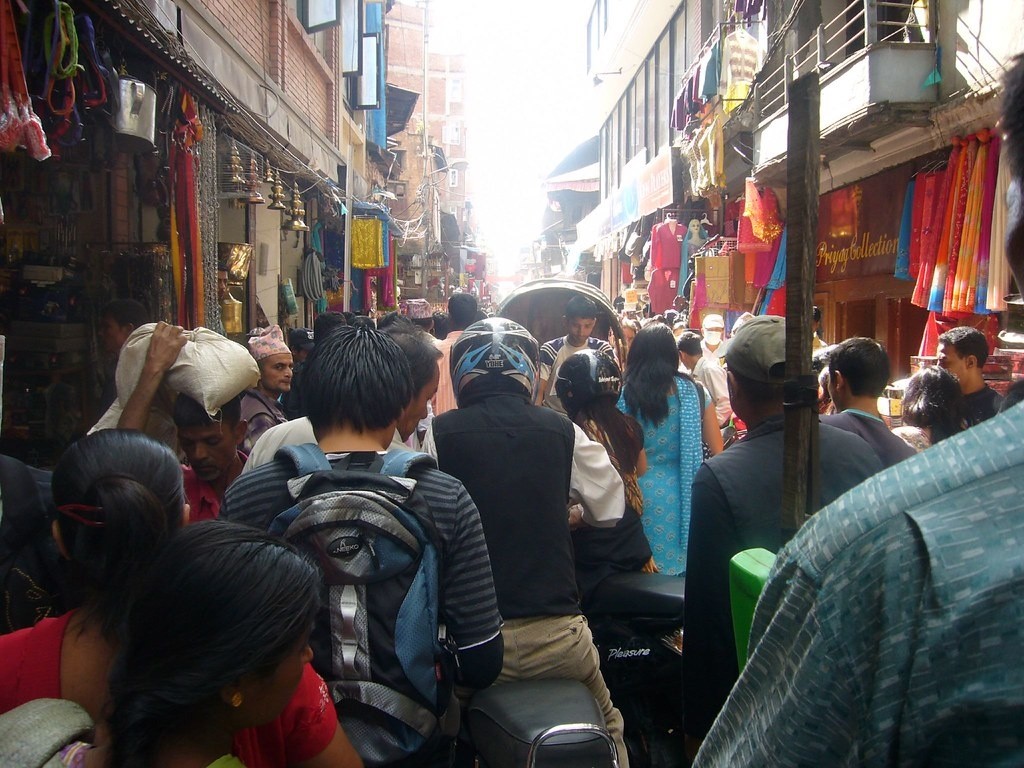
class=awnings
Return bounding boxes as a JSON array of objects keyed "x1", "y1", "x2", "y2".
[{"x1": 542, "y1": 162, "x2": 600, "y2": 211}]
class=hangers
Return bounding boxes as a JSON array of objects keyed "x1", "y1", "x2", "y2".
[
  {"x1": 680, "y1": 22, "x2": 760, "y2": 90},
  {"x1": 905, "y1": 157, "x2": 958, "y2": 176},
  {"x1": 677, "y1": 110, "x2": 719, "y2": 141},
  {"x1": 654, "y1": 213, "x2": 715, "y2": 227},
  {"x1": 352, "y1": 209, "x2": 381, "y2": 221}
]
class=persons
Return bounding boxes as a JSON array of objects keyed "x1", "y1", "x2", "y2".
[
  {"x1": 678, "y1": 220, "x2": 708, "y2": 296},
  {"x1": 616, "y1": 324, "x2": 723, "y2": 577},
  {"x1": 812, "y1": 305, "x2": 828, "y2": 349},
  {"x1": 676, "y1": 331, "x2": 733, "y2": 430},
  {"x1": 0, "y1": 263, "x2": 503, "y2": 767},
  {"x1": 891, "y1": 366, "x2": 960, "y2": 452},
  {"x1": 937, "y1": 327, "x2": 1002, "y2": 429},
  {"x1": 555, "y1": 350, "x2": 660, "y2": 575},
  {"x1": 700, "y1": 314, "x2": 724, "y2": 357},
  {"x1": 533, "y1": 294, "x2": 616, "y2": 411},
  {"x1": 680, "y1": 315, "x2": 884, "y2": 746},
  {"x1": 694, "y1": 52, "x2": 1024, "y2": 768},
  {"x1": 818, "y1": 338, "x2": 915, "y2": 465},
  {"x1": 418, "y1": 317, "x2": 631, "y2": 768},
  {"x1": 621, "y1": 309, "x2": 687, "y2": 346}
]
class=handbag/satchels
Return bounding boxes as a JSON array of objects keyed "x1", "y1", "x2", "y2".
[{"x1": 618, "y1": 208, "x2": 662, "y2": 284}]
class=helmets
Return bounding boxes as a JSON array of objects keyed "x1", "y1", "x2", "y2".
[
  {"x1": 450, "y1": 316, "x2": 540, "y2": 404},
  {"x1": 555, "y1": 348, "x2": 622, "y2": 406}
]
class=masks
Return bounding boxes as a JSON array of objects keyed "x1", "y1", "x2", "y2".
[{"x1": 703, "y1": 329, "x2": 723, "y2": 345}]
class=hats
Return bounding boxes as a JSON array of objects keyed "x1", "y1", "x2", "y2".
[
  {"x1": 247, "y1": 324, "x2": 291, "y2": 362},
  {"x1": 405, "y1": 299, "x2": 432, "y2": 319},
  {"x1": 281, "y1": 326, "x2": 315, "y2": 352},
  {"x1": 718, "y1": 314, "x2": 786, "y2": 384},
  {"x1": 702, "y1": 314, "x2": 725, "y2": 329}
]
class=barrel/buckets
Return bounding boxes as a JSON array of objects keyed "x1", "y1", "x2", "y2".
[{"x1": 112, "y1": 62, "x2": 159, "y2": 153}]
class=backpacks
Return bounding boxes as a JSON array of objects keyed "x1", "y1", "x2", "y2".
[{"x1": 268, "y1": 442, "x2": 457, "y2": 763}]
